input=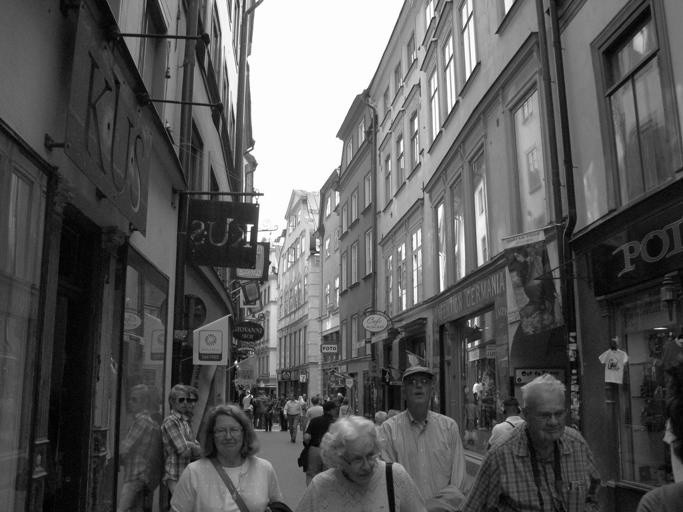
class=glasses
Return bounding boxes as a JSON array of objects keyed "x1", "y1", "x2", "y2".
[
  {"x1": 405, "y1": 379, "x2": 433, "y2": 385},
  {"x1": 179, "y1": 397, "x2": 198, "y2": 403}
]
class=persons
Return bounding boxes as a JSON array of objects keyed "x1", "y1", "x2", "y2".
[
  {"x1": 513, "y1": 246, "x2": 545, "y2": 304},
  {"x1": 294, "y1": 416, "x2": 428, "y2": 512},
  {"x1": 298, "y1": 401, "x2": 340, "y2": 491},
  {"x1": 637, "y1": 394, "x2": 683, "y2": 511},
  {"x1": 114, "y1": 384, "x2": 161, "y2": 512},
  {"x1": 456, "y1": 373, "x2": 603, "y2": 511},
  {"x1": 378, "y1": 364, "x2": 467, "y2": 511},
  {"x1": 187, "y1": 385, "x2": 201, "y2": 462},
  {"x1": 372, "y1": 410, "x2": 390, "y2": 438},
  {"x1": 486, "y1": 397, "x2": 527, "y2": 451},
  {"x1": 235, "y1": 386, "x2": 359, "y2": 443},
  {"x1": 160, "y1": 383, "x2": 202, "y2": 497},
  {"x1": 170, "y1": 403, "x2": 283, "y2": 512}
]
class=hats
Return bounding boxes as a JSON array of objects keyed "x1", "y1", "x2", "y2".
[
  {"x1": 323, "y1": 401, "x2": 340, "y2": 411},
  {"x1": 402, "y1": 366, "x2": 435, "y2": 384},
  {"x1": 504, "y1": 399, "x2": 519, "y2": 405}
]
